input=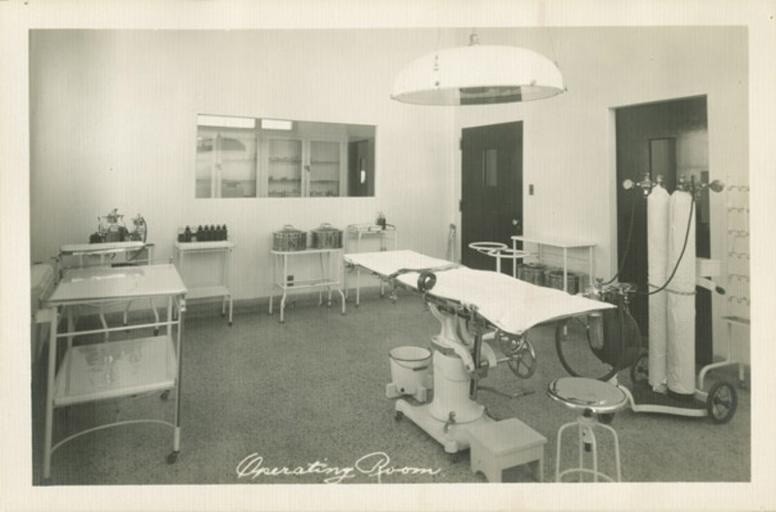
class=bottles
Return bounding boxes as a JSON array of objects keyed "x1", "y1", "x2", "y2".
[
  {"x1": 183, "y1": 224, "x2": 227, "y2": 242},
  {"x1": 287, "y1": 272, "x2": 294, "y2": 286}
]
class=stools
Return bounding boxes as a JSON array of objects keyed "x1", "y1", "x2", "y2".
[{"x1": 546, "y1": 378, "x2": 630, "y2": 482}]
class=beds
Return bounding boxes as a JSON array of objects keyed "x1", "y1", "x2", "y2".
[{"x1": 342, "y1": 249, "x2": 620, "y2": 455}]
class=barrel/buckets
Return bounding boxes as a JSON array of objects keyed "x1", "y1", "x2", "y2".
[
  {"x1": 272, "y1": 224, "x2": 307, "y2": 251},
  {"x1": 521, "y1": 263, "x2": 546, "y2": 285},
  {"x1": 310, "y1": 222, "x2": 342, "y2": 249},
  {"x1": 549, "y1": 269, "x2": 576, "y2": 295},
  {"x1": 388, "y1": 346, "x2": 432, "y2": 396}
]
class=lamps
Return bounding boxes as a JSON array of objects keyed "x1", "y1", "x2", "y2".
[{"x1": 389, "y1": 27, "x2": 565, "y2": 108}]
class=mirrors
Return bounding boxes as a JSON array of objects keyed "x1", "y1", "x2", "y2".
[{"x1": 194, "y1": 113, "x2": 376, "y2": 197}]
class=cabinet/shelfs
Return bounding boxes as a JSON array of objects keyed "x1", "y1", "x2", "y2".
[
  {"x1": 196, "y1": 128, "x2": 349, "y2": 196},
  {"x1": 44, "y1": 262, "x2": 189, "y2": 473},
  {"x1": 511, "y1": 234, "x2": 597, "y2": 335},
  {"x1": 171, "y1": 225, "x2": 399, "y2": 327}
]
